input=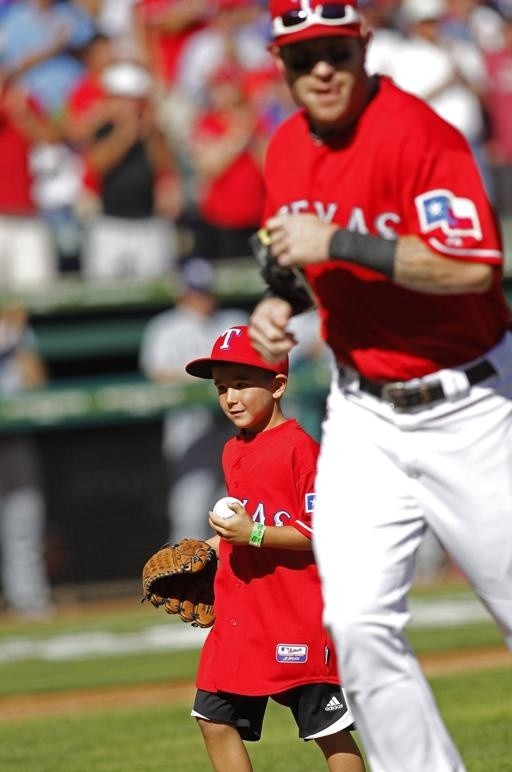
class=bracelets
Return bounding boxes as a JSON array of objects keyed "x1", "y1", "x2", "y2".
[
  {"x1": 249, "y1": 522, "x2": 266, "y2": 549},
  {"x1": 259, "y1": 285, "x2": 315, "y2": 318}
]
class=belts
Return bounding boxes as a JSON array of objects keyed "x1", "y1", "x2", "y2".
[{"x1": 359, "y1": 359, "x2": 498, "y2": 408}]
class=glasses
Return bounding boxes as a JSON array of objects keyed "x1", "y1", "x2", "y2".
[{"x1": 270, "y1": 2, "x2": 363, "y2": 37}]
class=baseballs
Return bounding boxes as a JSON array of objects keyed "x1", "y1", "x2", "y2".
[{"x1": 214, "y1": 497, "x2": 246, "y2": 520}]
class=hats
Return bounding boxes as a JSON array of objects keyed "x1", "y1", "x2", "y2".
[
  {"x1": 185, "y1": 325, "x2": 289, "y2": 379},
  {"x1": 266, "y1": 1, "x2": 363, "y2": 47}
]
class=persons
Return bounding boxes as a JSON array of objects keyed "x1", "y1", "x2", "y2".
[
  {"x1": 248, "y1": 0, "x2": 512, "y2": 772},
  {"x1": 1, "y1": 1, "x2": 512, "y2": 280},
  {"x1": 141, "y1": 258, "x2": 250, "y2": 548},
  {"x1": 277, "y1": 303, "x2": 332, "y2": 450},
  {"x1": 0, "y1": 289, "x2": 51, "y2": 615},
  {"x1": 142, "y1": 323, "x2": 365, "y2": 771}
]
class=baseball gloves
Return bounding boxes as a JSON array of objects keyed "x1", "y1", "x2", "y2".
[{"x1": 144, "y1": 538, "x2": 216, "y2": 628}]
